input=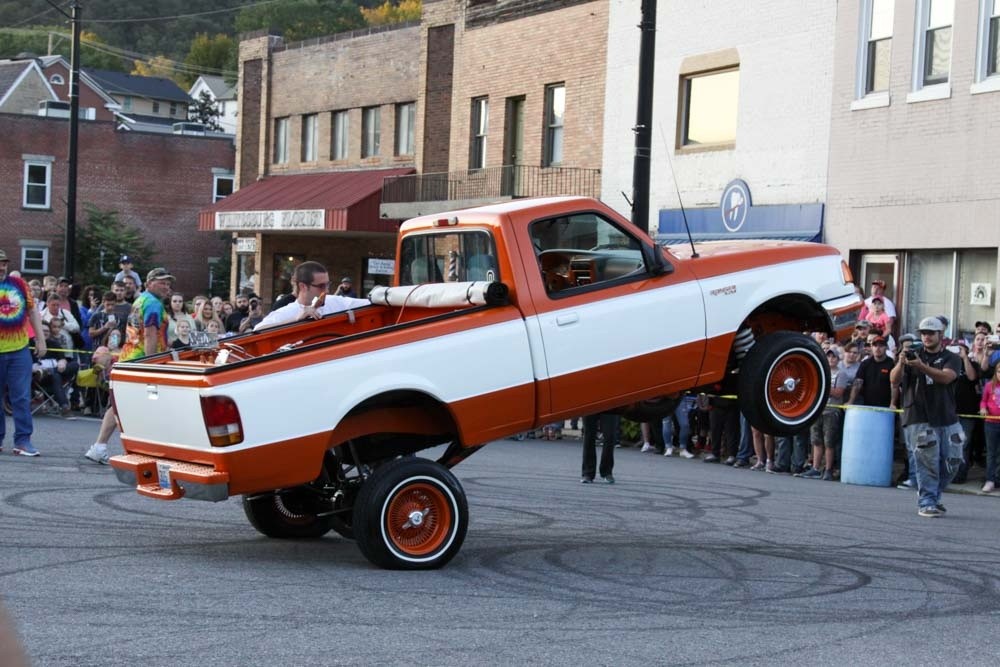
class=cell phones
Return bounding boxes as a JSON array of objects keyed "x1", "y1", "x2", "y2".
[
  {"x1": 108, "y1": 315, "x2": 115, "y2": 322},
  {"x1": 946, "y1": 345, "x2": 960, "y2": 354},
  {"x1": 111, "y1": 335, "x2": 117, "y2": 348},
  {"x1": 252, "y1": 304, "x2": 256, "y2": 310},
  {"x1": 992, "y1": 344, "x2": 1000, "y2": 350}
]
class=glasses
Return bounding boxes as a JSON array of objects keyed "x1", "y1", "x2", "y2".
[{"x1": 302, "y1": 281, "x2": 332, "y2": 289}]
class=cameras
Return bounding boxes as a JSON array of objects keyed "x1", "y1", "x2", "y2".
[{"x1": 905, "y1": 349, "x2": 921, "y2": 360}]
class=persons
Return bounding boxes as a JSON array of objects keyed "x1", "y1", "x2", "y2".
[
  {"x1": 890, "y1": 317, "x2": 966, "y2": 517},
  {"x1": 0, "y1": 250, "x2": 47, "y2": 456},
  {"x1": 502, "y1": 280, "x2": 1000, "y2": 493},
  {"x1": 84, "y1": 267, "x2": 176, "y2": 465},
  {"x1": 0, "y1": 254, "x2": 371, "y2": 420}
]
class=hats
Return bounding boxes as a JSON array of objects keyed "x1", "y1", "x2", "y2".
[
  {"x1": 856, "y1": 320, "x2": 872, "y2": 329},
  {"x1": 871, "y1": 295, "x2": 884, "y2": 303},
  {"x1": 916, "y1": 317, "x2": 944, "y2": 331},
  {"x1": 935, "y1": 317, "x2": 948, "y2": 325},
  {"x1": 975, "y1": 321, "x2": 991, "y2": 333},
  {"x1": 872, "y1": 336, "x2": 887, "y2": 344},
  {"x1": 56, "y1": 276, "x2": 69, "y2": 282},
  {"x1": 147, "y1": 267, "x2": 176, "y2": 283},
  {"x1": 824, "y1": 348, "x2": 839, "y2": 356},
  {"x1": 898, "y1": 333, "x2": 918, "y2": 342},
  {"x1": 119, "y1": 255, "x2": 134, "y2": 263},
  {"x1": 0, "y1": 250, "x2": 8, "y2": 261},
  {"x1": 341, "y1": 277, "x2": 352, "y2": 283},
  {"x1": 871, "y1": 279, "x2": 887, "y2": 288}
]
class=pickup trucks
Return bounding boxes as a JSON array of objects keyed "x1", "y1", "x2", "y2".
[{"x1": 107, "y1": 192, "x2": 865, "y2": 573}]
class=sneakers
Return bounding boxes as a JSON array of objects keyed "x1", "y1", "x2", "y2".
[
  {"x1": 918, "y1": 504, "x2": 946, "y2": 517},
  {"x1": 13, "y1": 442, "x2": 40, "y2": 456},
  {"x1": 85, "y1": 445, "x2": 111, "y2": 465}
]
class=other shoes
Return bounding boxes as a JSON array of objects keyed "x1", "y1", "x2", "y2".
[
  {"x1": 604, "y1": 475, "x2": 615, "y2": 484},
  {"x1": 61, "y1": 402, "x2": 92, "y2": 420},
  {"x1": 580, "y1": 476, "x2": 593, "y2": 484},
  {"x1": 639, "y1": 441, "x2": 834, "y2": 480},
  {"x1": 981, "y1": 481, "x2": 995, "y2": 492}
]
